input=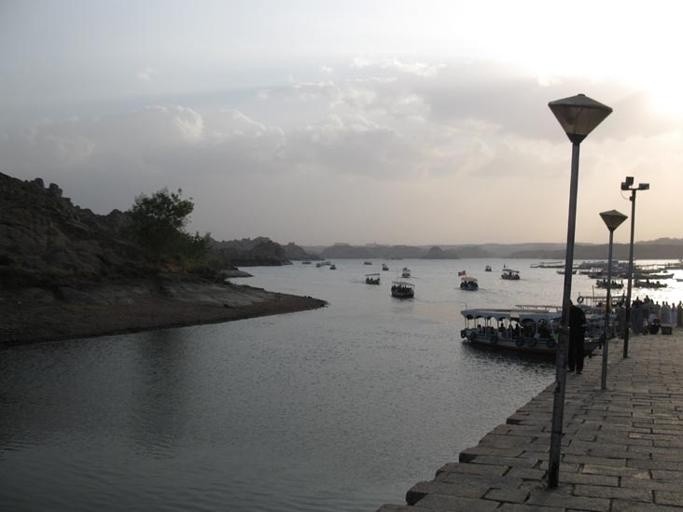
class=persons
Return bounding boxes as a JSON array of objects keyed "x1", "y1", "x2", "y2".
[
  {"x1": 499, "y1": 322, "x2": 506, "y2": 330},
  {"x1": 567, "y1": 299, "x2": 588, "y2": 376},
  {"x1": 515, "y1": 324, "x2": 522, "y2": 332}
]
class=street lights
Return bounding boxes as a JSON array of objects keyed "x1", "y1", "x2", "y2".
[
  {"x1": 599, "y1": 209, "x2": 628, "y2": 388},
  {"x1": 620, "y1": 175, "x2": 653, "y2": 358},
  {"x1": 545, "y1": 91, "x2": 614, "y2": 492}
]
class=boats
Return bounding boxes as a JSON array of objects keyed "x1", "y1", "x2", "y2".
[
  {"x1": 501, "y1": 268, "x2": 520, "y2": 280},
  {"x1": 458, "y1": 292, "x2": 634, "y2": 358},
  {"x1": 363, "y1": 259, "x2": 416, "y2": 300},
  {"x1": 604, "y1": 285, "x2": 605, "y2": 285},
  {"x1": 458, "y1": 270, "x2": 479, "y2": 291},
  {"x1": 485, "y1": 265, "x2": 492, "y2": 272},
  {"x1": 530, "y1": 257, "x2": 682, "y2": 290},
  {"x1": 301, "y1": 260, "x2": 336, "y2": 270}
]
